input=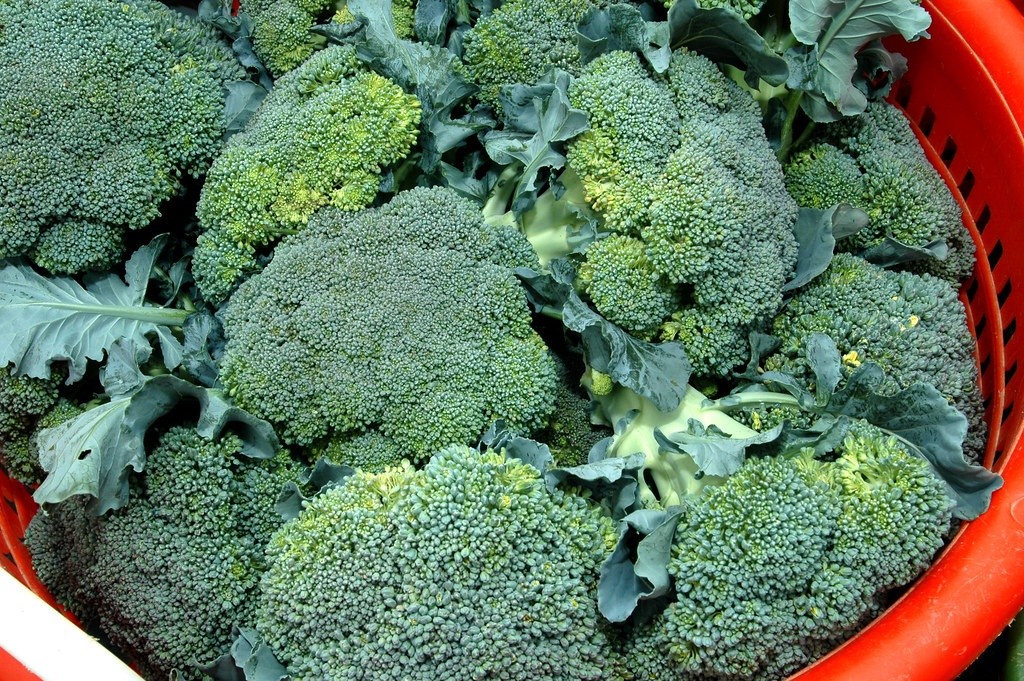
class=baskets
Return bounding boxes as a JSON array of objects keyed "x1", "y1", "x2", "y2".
[{"x1": 0, "y1": 0, "x2": 1024, "y2": 681}]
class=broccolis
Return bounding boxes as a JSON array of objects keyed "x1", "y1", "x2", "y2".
[{"x1": 0, "y1": 0, "x2": 1004, "y2": 681}]
